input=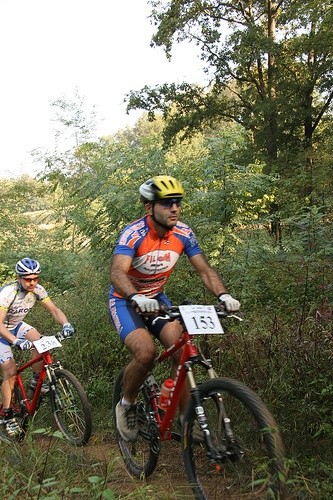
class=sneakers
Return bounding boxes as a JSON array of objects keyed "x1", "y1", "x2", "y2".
[
  {"x1": 176, "y1": 415, "x2": 204, "y2": 442},
  {"x1": 28, "y1": 378, "x2": 49, "y2": 393},
  {"x1": 115, "y1": 399, "x2": 138, "y2": 441},
  {"x1": 0, "y1": 416, "x2": 21, "y2": 437}
]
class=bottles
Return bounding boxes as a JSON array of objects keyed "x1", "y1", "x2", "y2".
[
  {"x1": 159, "y1": 379, "x2": 174, "y2": 409},
  {"x1": 26, "y1": 374, "x2": 39, "y2": 397}
]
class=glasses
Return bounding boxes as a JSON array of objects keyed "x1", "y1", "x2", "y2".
[
  {"x1": 158, "y1": 198, "x2": 181, "y2": 207},
  {"x1": 20, "y1": 277, "x2": 38, "y2": 283}
]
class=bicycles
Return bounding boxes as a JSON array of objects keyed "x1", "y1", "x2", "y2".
[
  {"x1": 111, "y1": 300, "x2": 289, "y2": 500},
  {"x1": 0, "y1": 329, "x2": 93, "y2": 447}
]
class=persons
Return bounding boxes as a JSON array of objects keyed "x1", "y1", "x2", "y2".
[
  {"x1": 109, "y1": 175, "x2": 242, "y2": 440},
  {"x1": 0, "y1": 256, "x2": 74, "y2": 438}
]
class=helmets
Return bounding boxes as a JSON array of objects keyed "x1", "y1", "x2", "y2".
[
  {"x1": 15, "y1": 258, "x2": 41, "y2": 276},
  {"x1": 138, "y1": 175, "x2": 184, "y2": 201}
]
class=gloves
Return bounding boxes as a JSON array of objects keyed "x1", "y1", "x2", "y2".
[
  {"x1": 12, "y1": 339, "x2": 31, "y2": 350},
  {"x1": 126, "y1": 293, "x2": 159, "y2": 316},
  {"x1": 62, "y1": 323, "x2": 74, "y2": 338},
  {"x1": 216, "y1": 292, "x2": 239, "y2": 312}
]
